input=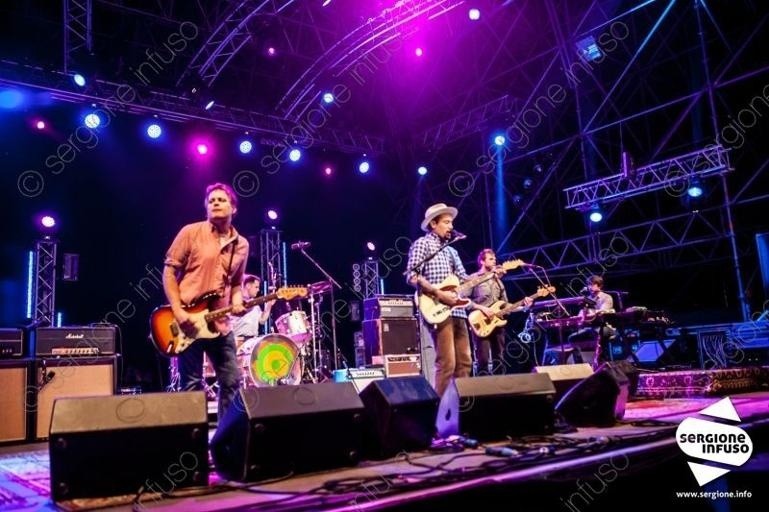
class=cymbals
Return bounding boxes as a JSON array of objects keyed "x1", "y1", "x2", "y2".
[{"x1": 307, "y1": 281, "x2": 332, "y2": 294}]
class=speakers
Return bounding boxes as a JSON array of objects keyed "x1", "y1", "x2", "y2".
[
  {"x1": 347, "y1": 263, "x2": 364, "y2": 294},
  {"x1": 530, "y1": 362, "x2": 595, "y2": 401},
  {"x1": 361, "y1": 317, "x2": 421, "y2": 368},
  {"x1": 556, "y1": 359, "x2": 630, "y2": 427},
  {"x1": 210, "y1": 381, "x2": 366, "y2": 483},
  {"x1": 47, "y1": 389, "x2": 208, "y2": 501},
  {"x1": 358, "y1": 377, "x2": 440, "y2": 461},
  {"x1": 436, "y1": 372, "x2": 557, "y2": 440},
  {"x1": 652, "y1": 331, "x2": 730, "y2": 369}
]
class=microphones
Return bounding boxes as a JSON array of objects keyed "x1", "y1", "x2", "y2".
[
  {"x1": 577, "y1": 286, "x2": 588, "y2": 294},
  {"x1": 291, "y1": 242, "x2": 311, "y2": 251},
  {"x1": 451, "y1": 229, "x2": 466, "y2": 242},
  {"x1": 520, "y1": 263, "x2": 542, "y2": 269}
]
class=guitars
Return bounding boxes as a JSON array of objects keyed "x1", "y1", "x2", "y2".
[
  {"x1": 414, "y1": 258, "x2": 526, "y2": 328},
  {"x1": 149, "y1": 284, "x2": 312, "y2": 357},
  {"x1": 467, "y1": 284, "x2": 557, "y2": 337}
]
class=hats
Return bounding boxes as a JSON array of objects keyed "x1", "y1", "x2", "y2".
[{"x1": 421, "y1": 203, "x2": 458, "y2": 231}]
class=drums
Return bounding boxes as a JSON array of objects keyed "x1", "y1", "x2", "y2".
[
  {"x1": 169, "y1": 351, "x2": 216, "y2": 378},
  {"x1": 234, "y1": 334, "x2": 304, "y2": 388},
  {"x1": 274, "y1": 310, "x2": 313, "y2": 346}
]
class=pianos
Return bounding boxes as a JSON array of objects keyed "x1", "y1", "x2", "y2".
[
  {"x1": 528, "y1": 296, "x2": 597, "y2": 309},
  {"x1": 534, "y1": 308, "x2": 677, "y2": 331}
]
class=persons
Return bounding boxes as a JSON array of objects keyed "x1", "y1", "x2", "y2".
[
  {"x1": 406, "y1": 202, "x2": 508, "y2": 398},
  {"x1": 569, "y1": 276, "x2": 614, "y2": 365},
  {"x1": 228, "y1": 275, "x2": 278, "y2": 343},
  {"x1": 464, "y1": 248, "x2": 535, "y2": 376},
  {"x1": 161, "y1": 182, "x2": 253, "y2": 428}
]
